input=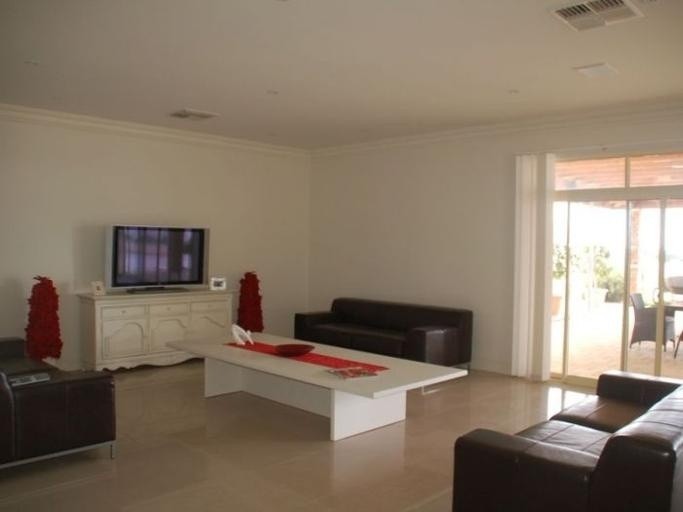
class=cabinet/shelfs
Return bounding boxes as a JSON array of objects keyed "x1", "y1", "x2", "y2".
[{"x1": 76, "y1": 290, "x2": 234, "y2": 373}]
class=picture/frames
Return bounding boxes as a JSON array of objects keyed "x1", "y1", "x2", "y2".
[
  {"x1": 208, "y1": 277, "x2": 227, "y2": 291},
  {"x1": 91, "y1": 280, "x2": 105, "y2": 296}
]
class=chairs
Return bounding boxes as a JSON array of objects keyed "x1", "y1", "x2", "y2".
[{"x1": 628, "y1": 290, "x2": 674, "y2": 352}]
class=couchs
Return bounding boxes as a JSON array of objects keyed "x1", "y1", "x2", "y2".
[
  {"x1": 451, "y1": 368, "x2": 682, "y2": 512},
  {"x1": 0, "y1": 336, "x2": 117, "y2": 470},
  {"x1": 293, "y1": 298, "x2": 472, "y2": 396}
]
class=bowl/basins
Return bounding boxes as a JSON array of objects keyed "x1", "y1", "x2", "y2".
[{"x1": 274, "y1": 344, "x2": 314, "y2": 356}]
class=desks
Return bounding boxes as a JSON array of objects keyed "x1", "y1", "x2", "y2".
[{"x1": 661, "y1": 299, "x2": 682, "y2": 359}]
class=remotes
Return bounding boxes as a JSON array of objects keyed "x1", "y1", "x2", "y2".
[{"x1": 8, "y1": 371, "x2": 51, "y2": 386}]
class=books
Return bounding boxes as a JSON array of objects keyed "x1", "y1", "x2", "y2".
[{"x1": 325, "y1": 368, "x2": 378, "y2": 380}]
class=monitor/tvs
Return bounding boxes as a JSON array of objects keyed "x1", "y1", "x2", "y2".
[{"x1": 105, "y1": 223, "x2": 211, "y2": 295}]
